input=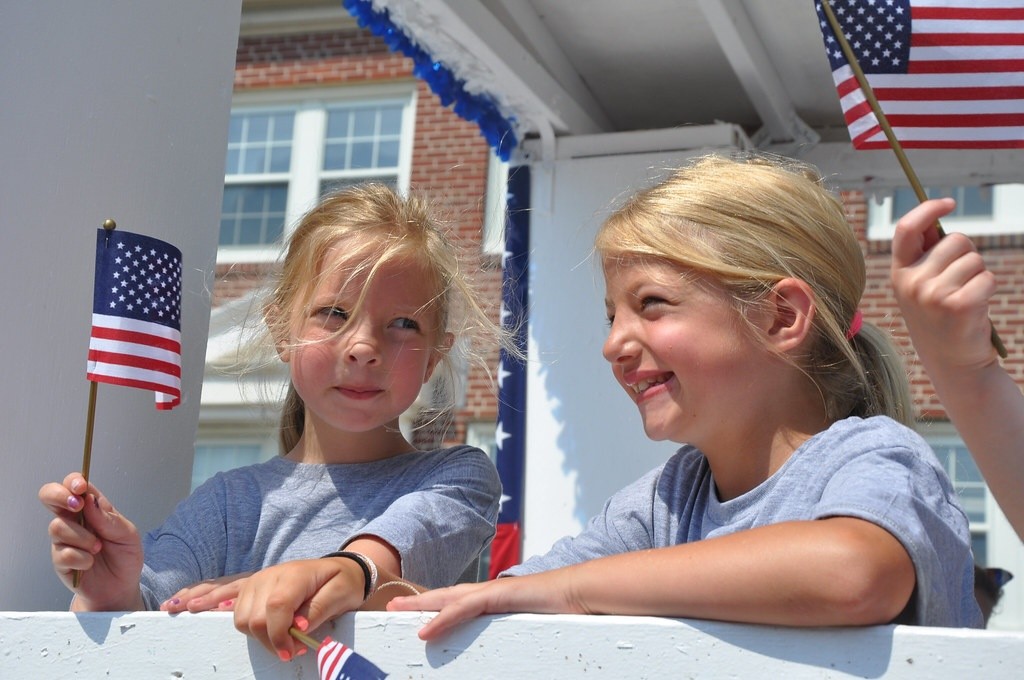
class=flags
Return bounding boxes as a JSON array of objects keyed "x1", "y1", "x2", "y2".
[
  {"x1": 315, "y1": 638, "x2": 388, "y2": 680},
  {"x1": 86, "y1": 229, "x2": 183, "y2": 409},
  {"x1": 814, "y1": 0, "x2": 1024, "y2": 149}
]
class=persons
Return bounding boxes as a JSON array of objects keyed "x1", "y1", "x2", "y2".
[
  {"x1": 233, "y1": 156, "x2": 983, "y2": 660},
  {"x1": 891, "y1": 197, "x2": 1024, "y2": 541},
  {"x1": 39, "y1": 186, "x2": 503, "y2": 613}
]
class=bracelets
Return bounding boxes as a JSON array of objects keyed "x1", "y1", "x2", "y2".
[{"x1": 321, "y1": 551, "x2": 377, "y2": 601}]
